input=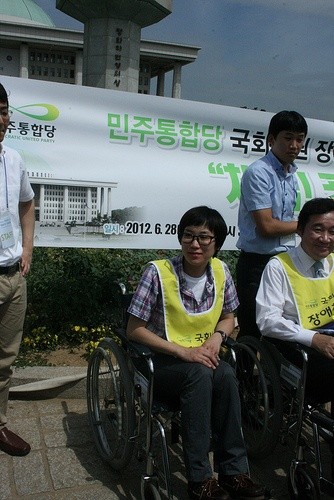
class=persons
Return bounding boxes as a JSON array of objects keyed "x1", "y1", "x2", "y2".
[
  {"x1": 0, "y1": 83, "x2": 35, "y2": 457},
  {"x1": 126, "y1": 206, "x2": 265, "y2": 500},
  {"x1": 236, "y1": 110, "x2": 308, "y2": 351},
  {"x1": 256, "y1": 198, "x2": 334, "y2": 419}
]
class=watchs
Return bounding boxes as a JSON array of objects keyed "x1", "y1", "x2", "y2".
[{"x1": 214, "y1": 330, "x2": 228, "y2": 346}]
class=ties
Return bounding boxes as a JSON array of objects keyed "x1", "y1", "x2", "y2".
[{"x1": 313, "y1": 261, "x2": 328, "y2": 278}]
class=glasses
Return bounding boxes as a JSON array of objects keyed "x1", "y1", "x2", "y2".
[
  {"x1": 0, "y1": 110, "x2": 13, "y2": 119},
  {"x1": 180, "y1": 232, "x2": 215, "y2": 246}
]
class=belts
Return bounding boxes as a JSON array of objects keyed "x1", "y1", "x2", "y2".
[{"x1": 0, "y1": 260, "x2": 22, "y2": 277}]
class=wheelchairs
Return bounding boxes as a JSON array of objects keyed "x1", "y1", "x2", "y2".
[{"x1": 85, "y1": 283, "x2": 334, "y2": 500}]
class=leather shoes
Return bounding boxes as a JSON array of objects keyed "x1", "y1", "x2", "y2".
[
  {"x1": 0, "y1": 427, "x2": 31, "y2": 456},
  {"x1": 218, "y1": 474, "x2": 264, "y2": 500},
  {"x1": 188, "y1": 477, "x2": 231, "y2": 500}
]
